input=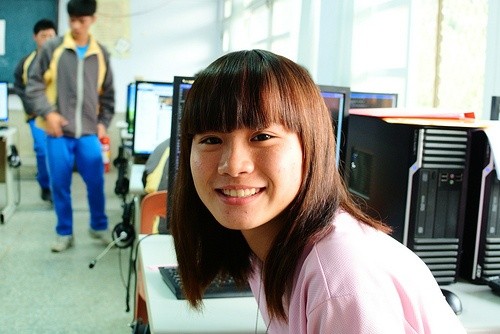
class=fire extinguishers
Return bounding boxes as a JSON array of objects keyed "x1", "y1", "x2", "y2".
[{"x1": 99, "y1": 134, "x2": 111, "y2": 174}]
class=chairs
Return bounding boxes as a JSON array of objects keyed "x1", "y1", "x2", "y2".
[{"x1": 134, "y1": 190, "x2": 173, "y2": 334}]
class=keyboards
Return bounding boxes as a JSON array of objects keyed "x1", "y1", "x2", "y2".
[{"x1": 158, "y1": 266, "x2": 255, "y2": 299}]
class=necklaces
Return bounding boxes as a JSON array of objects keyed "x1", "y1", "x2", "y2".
[{"x1": 254, "y1": 261, "x2": 275, "y2": 334}]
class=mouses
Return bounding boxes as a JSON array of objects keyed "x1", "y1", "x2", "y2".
[{"x1": 440, "y1": 289, "x2": 462, "y2": 314}]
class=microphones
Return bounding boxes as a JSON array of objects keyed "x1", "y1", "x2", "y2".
[{"x1": 89, "y1": 232, "x2": 128, "y2": 269}]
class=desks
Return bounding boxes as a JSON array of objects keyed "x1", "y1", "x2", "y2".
[
  {"x1": 121, "y1": 128, "x2": 134, "y2": 146},
  {"x1": 136, "y1": 234, "x2": 500, "y2": 334},
  {"x1": 129, "y1": 163, "x2": 146, "y2": 261},
  {"x1": 117, "y1": 120, "x2": 129, "y2": 128},
  {"x1": 0, "y1": 127, "x2": 20, "y2": 225}
]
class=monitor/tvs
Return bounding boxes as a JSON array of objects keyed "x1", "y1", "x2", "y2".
[
  {"x1": 127, "y1": 76, "x2": 398, "y2": 232},
  {"x1": 0, "y1": 81, "x2": 8, "y2": 121}
]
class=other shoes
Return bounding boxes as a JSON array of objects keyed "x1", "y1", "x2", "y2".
[
  {"x1": 87, "y1": 227, "x2": 116, "y2": 248},
  {"x1": 41, "y1": 188, "x2": 50, "y2": 201},
  {"x1": 51, "y1": 235, "x2": 73, "y2": 252}
]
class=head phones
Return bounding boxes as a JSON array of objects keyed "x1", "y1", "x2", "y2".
[
  {"x1": 8, "y1": 146, "x2": 21, "y2": 168},
  {"x1": 111, "y1": 146, "x2": 137, "y2": 249}
]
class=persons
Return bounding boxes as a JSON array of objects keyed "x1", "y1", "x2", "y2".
[
  {"x1": 11, "y1": 18, "x2": 58, "y2": 206},
  {"x1": 24, "y1": 0, "x2": 119, "y2": 253},
  {"x1": 169, "y1": 49, "x2": 469, "y2": 334}
]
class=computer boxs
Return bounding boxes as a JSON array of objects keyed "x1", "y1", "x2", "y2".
[{"x1": 342, "y1": 115, "x2": 500, "y2": 286}]
